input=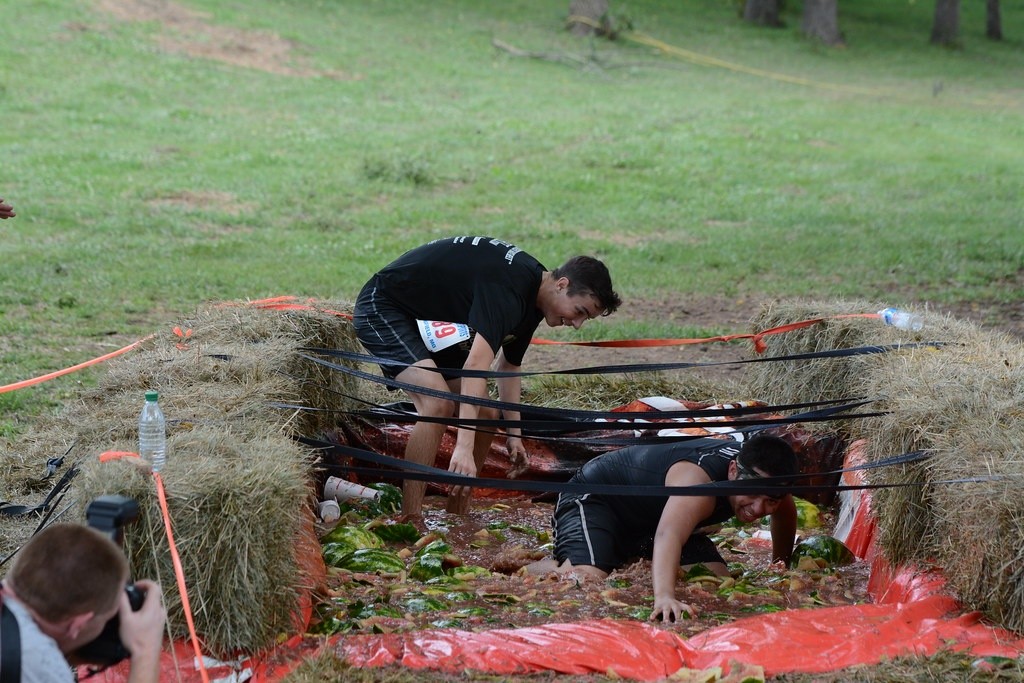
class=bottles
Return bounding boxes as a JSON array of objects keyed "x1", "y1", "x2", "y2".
[
  {"x1": 876, "y1": 308, "x2": 923, "y2": 333},
  {"x1": 139, "y1": 392, "x2": 167, "y2": 473}
]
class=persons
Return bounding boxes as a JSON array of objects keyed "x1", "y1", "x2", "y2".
[
  {"x1": 0, "y1": 524, "x2": 167, "y2": 683},
  {"x1": 352, "y1": 234, "x2": 622, "y2": 538},
  {"x1": 519, "y1": 432, "x2": 805, "y2": 624}
]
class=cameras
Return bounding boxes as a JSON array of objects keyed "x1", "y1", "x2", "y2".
[{"x1": 66, "y1": 493, "x2": 145, "y2": 668}]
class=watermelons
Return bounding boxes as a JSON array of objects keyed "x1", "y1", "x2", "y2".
[{"x1": 321, "y1": 480, "x2": 854, "y2": 621}]
